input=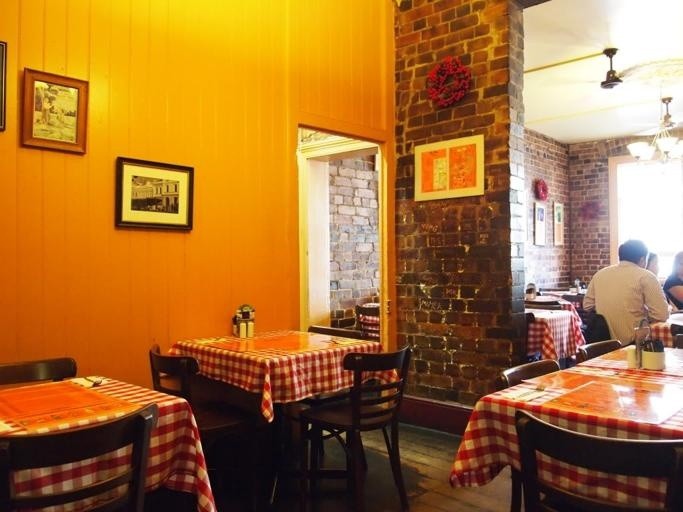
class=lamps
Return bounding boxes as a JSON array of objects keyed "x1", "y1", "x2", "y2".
[{"x1": 626, "y1": 97, "x2": 683, "y2": 164}]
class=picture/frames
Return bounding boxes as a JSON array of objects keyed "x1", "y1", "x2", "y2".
[
  {"x1": 21, "y1": 69, "x2": 89, "y2": 156},
  {"x1": 114, "y1": 156, "x2": 194, "y2": 232},
  {"x1": 534, "y1": 202, "x2": 547, "y2": 247},
  {"x1": 0, "y1": 41, "x2": 6, "y2": 131},
  {"x1": 414, "y1": 134, "x2": 484, "y2": 202},
  {"x1": 554, "y1": 203, "x2": 565, "y2": 247}
]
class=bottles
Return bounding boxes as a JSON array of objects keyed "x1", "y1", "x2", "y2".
[
  {"x1": 574, "y1": 276, "x2": 580, "y2": 286},
  {"x1": 249, "y1": 309, "x2": 255, "y2": 319},
  {"x1": 236, "y1": 310, "x2": 242, "y2": 320}
]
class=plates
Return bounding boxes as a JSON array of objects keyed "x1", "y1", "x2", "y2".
[{"x1": 534, "y1": 295, "x2": 561, "y2": 303}]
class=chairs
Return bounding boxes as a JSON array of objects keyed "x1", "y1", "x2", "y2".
[
  {"x1": 0, "y1": 358, "x2": 78, "y2": 388},
  {"x1": 303, "y1": 326, "x2": 362, "y2": 468},
  {"x1": 1, "y1": 402, "x2": 153, "y2": 511},
  {"x1": 499, "y1": 359, "x2": 561, "y2": 512},
  {"x1": 353, "y1": 305, "x2": 384, "y2": 341},
  {"x1": 576, "y1": 339, "x2": 622, "y2": 365},
  {"x1": 148, "y1": 346, "x2": 262, "y2": 511},
  {"x1": 514, "y1": 411, "x2": 683, "y2": 512},
  {"x1": 300, "y1": 343, "x2": 408, "y2": 512}
]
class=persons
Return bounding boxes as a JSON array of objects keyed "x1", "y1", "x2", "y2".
[
  {"x1": 663, "y1": 251, "x2": 683, "y2": 311},
  {"x1": 645, "y1": 254, "x2": 659, "y2": 278},
  {"x1": 583, "y1": 240, "x2": 670, "y2": 344}
]
